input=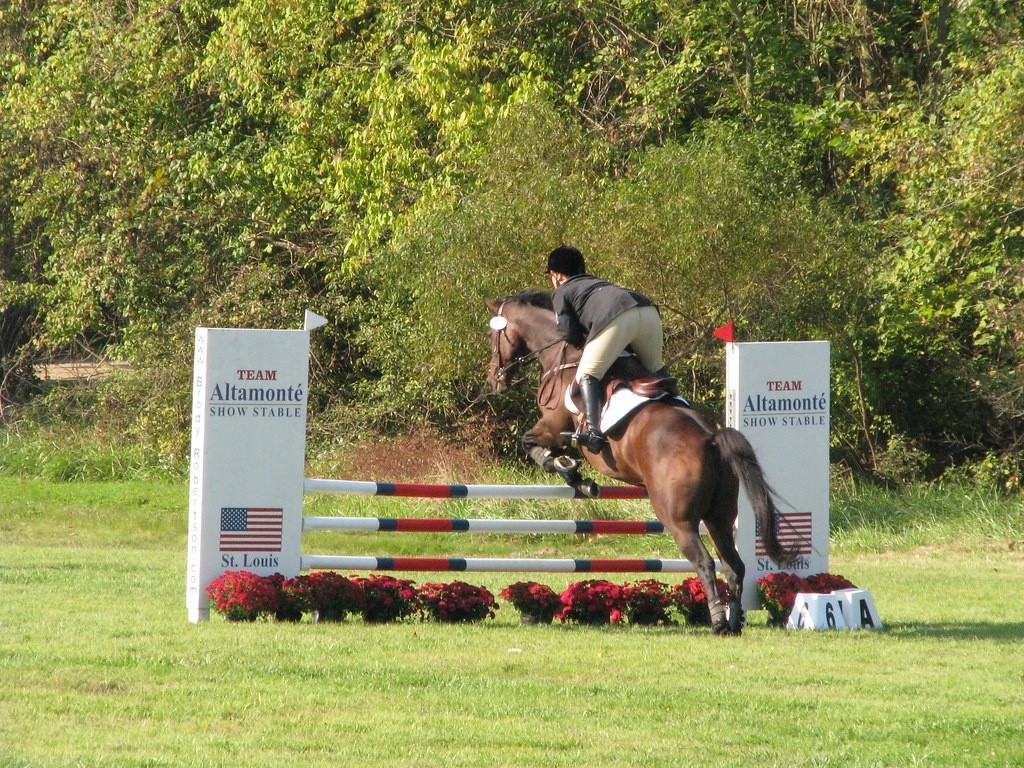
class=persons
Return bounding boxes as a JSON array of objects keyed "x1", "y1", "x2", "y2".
[{"x1": 542, "y1": 245, "x2": 687, "y2": 449}]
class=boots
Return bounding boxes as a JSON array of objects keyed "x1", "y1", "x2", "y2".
[
  {"x1": 655, "y1": 366, "x2": 670, "y2": 378},
  {"x1": 559, "y1": 374, "x2": 604, "y2": 455}
]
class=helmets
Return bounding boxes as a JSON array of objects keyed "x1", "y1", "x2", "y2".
[{"x1": 542, "y1": 246, "x2": 586, "y2": 277}]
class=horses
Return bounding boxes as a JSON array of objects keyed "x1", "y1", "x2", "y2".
[{"x1": 481, "y1": 291, "x2": 802, "y2": 635}]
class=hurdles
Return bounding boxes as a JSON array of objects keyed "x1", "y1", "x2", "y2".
[{"x1": 184, "y1": 326, "x2": 832, "y2": 626}]
